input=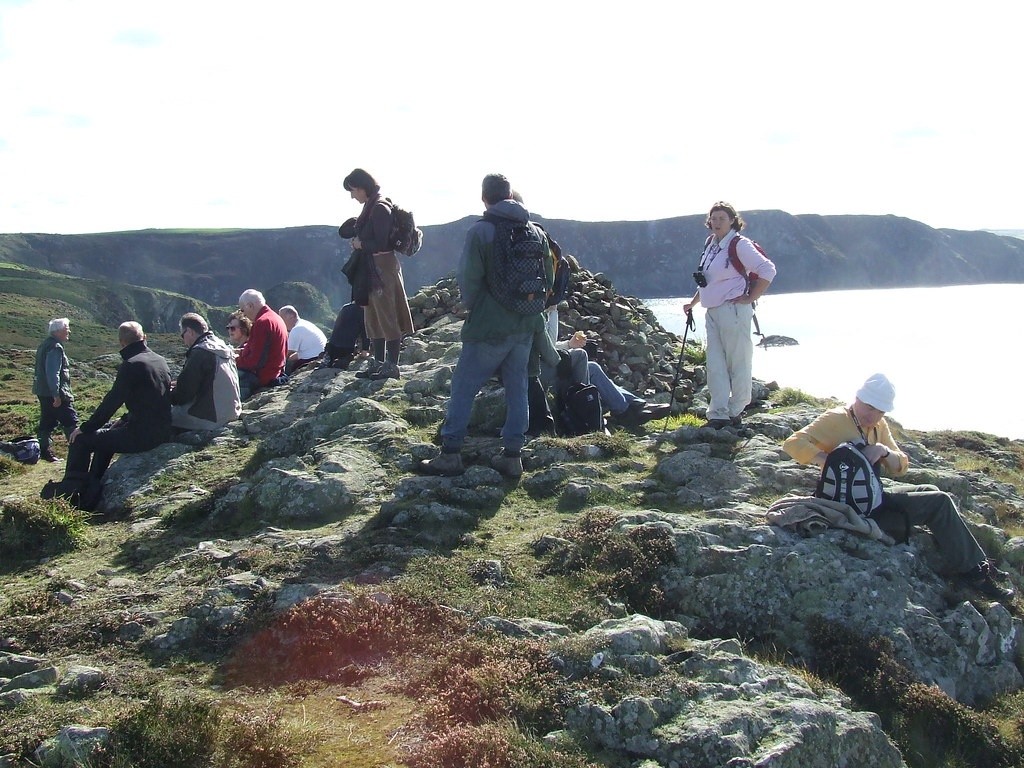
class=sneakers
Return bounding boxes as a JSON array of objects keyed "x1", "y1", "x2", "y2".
[
  {"x1": 370, "y1": 361, "x2": 400, "y2": 380},
  {"x1": 491, "y1": 450, "x2": 523, "y2": 477},
  {"x1": 971, "y1": 567, "x2": 1013, "y2": 601},
  {"x1": 983, "y1": 556, "x2": 1010, "y2": 582},
  {"x1": 420, "y1": 449, "x2": 465, "y2": 475},
  {"x1": 354, "y1": 360, "x2": 384, "y2": 379}
]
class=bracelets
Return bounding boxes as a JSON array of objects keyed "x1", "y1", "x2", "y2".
[{"x1": 881, "y1": 446, "x2": 891, "y2": 458}]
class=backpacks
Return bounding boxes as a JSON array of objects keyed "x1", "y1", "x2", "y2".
[
  {"x1": 375, "y1": 199, "x2": 423, "y2": 256},
  {"x1": 553, "y1": 383, "x2": 603, "y2": 436},
  {"x1": 475, "y1": 216, "x2": 546, "y2": 315},
  {"x1": 810, "y1": 441, "x2": 883, "y2": 517},
  {"x1": 706, "y1": 235, "x2": 766, "y2": 286},
  {"x1": 546, "y1": 236, "x2": 571, "y2": 308}
]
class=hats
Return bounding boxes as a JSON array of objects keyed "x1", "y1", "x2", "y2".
[{"x1": 856, "y1": 373, "x2": 895, "y2": 412}]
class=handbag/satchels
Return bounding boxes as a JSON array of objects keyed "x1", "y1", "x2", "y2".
[{"x1": 9, "y1": 436, "x2": 40, "y2": 464}]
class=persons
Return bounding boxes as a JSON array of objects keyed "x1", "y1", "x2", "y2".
[
  {"x1": 683, "y1": 201, "x2": 777, "y2": 431},
  {"x1": 168, "y1": 312, "x2": 242, "y2": 448},
  {"x1": 225, "y1": 309, "x2": 253, "y2": 357},
  {"x1": 279, "y1": 305, "x2": 329, "y2": 377},
  {"x1": 307, "y1": 301, "x2": 371, "y2": 371},
  {"x1": 419, "y1": 174, "x2": 671, "y2": 479},
  {"x1": 49, "y1": 320, "x2": 171, "y2": 509},
  {"x1": 32, "y1": 317, "x2": 80, "y2": 463},
  {"x1": 232, "y1": 289, "x2": 288, "y2": 402},
  {"x1": 344, "y1": 168, "x2": 416, "y2": 381},
  {"x1": 782, "y1": 373, "x2": 1015, "y2": 603}
]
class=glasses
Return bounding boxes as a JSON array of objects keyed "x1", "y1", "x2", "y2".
[
  {"x1": 226, "y1": 326, "x2": 242, "y2": 332},
  {"x1": 240, "y1": 304, "x2": 246, "y2": 312},
  {"x1": 182, "y1": 328, "x2": 189, "y2": 338}
]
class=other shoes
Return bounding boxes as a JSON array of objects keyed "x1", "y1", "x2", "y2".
[
  {"x1": 333, "y1": 353, "x2": 354, "y2": 369},
  {"x1": 728, "y1": 413, "x2": 742, "y2": 425},
  {"x1": 700, "y1": 419, "x2": 730, "y2": 431},
  {"x1": 639, "y1": 402, "x2": 671, "y2": 421},
  {"x1": 614, "y1": 405, "x2": 654, "y2": 428},
  {"x1": 45, "y1": 449, "x2": 59, "y2": 461},
  {"x1": 319, "y1": 353, "x2": 334, "y2": 368},
  {"x1": 43, "y1": 480, "x2": 96, "y2": 512}
]
